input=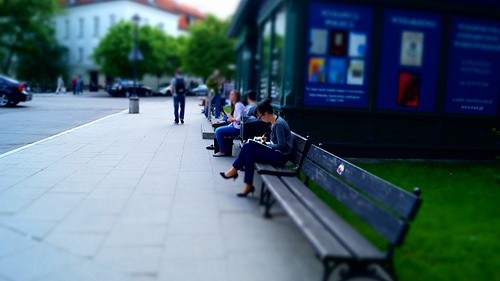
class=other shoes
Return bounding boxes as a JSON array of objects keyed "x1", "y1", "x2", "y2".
[
  {"x1": 206, "y1": 144, "x2": 215, "y2": 150},
  {"x1": 213, "y1": 152, "x2": 227, "y2": 157}
]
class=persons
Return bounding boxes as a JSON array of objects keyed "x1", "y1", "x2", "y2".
[
  {"x1": 206, "y1": 89, "x2": 238, "y2": 149},
  {"x1": 169, "y1": 69, "x2": 187, "y2": 123},
  {"x1": 197, "y1": 81, "x2": 224, "y2": 110},
  {"x1": 212, "y1": 90, "x2": 259, "y2": 156},
  {"x1": 219, "y1": 97, "x2": 294, "y2": 195},
  {"x1": 52, "y1": 70, "x2": 89, "y2": 95}
]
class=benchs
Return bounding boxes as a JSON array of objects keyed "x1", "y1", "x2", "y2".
[
  {"x1": 239, "y1": 109, "x2": 279, "y2": 147},
  {"x1": 261, "y1": 143, "x2": 423, "y2": 281},
  {"x1": 239, "y1": 130, "x2": 309, "y2": 206}
]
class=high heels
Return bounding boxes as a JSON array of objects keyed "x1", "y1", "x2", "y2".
[
  {"x1": 237, "y1": 186, "x2": 255, "y2": 197},
  {"x1": 174, "y1": 120, "x2": 184, "y2": 125},
  {"x1": 219, "y1": 171, "x2": 238, "y2": 182}
]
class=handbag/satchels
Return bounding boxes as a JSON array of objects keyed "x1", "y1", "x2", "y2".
[
  {"x1": 214, "y1": 137, "x2": 232, "y2": 156},
  {"x1": 211, "y1": 120, "x2": 226, "y2": 127}
]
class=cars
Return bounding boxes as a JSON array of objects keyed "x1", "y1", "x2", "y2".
[
  {"x1": 107, "y1": 80, "x2": 153, "y2": 98},
  {"x1": 152, "y1": 82, "x2": 174, "y2": 95},
  {"x1": 188, "y1": 83, "x2": 208, "y2": 96},
  {"x1": 0, "y1": 74, "x2": 32, "y2": 107}
]
